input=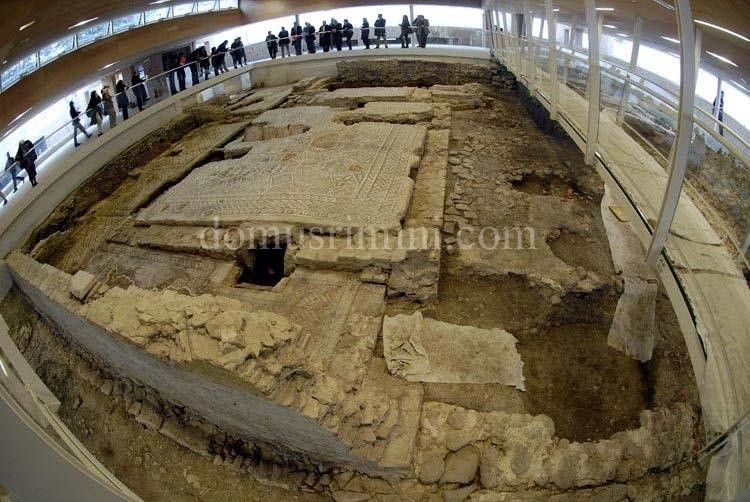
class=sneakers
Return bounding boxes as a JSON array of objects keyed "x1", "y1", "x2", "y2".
[
  {"x1": 32, "y1": 182, "x2": 37, "y2": 187},
  {"x1": 3, "y1": 199, "x2": 7, "y2": 206},
  {"x1": 87, "y1": 133, "x2": 92, "y2": 138},
  {"x1": 21, "y1": 176, "x2": 25, "y2": 183},
  {"x1": 13, "y1": 188, "x2": 17, "y2": 193},
  {"x1": 74, "y1": 142, "x2": 81, "y2": 147}
]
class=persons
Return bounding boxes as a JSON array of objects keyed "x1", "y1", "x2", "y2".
[
  {"x1": 0, "y1": 139, "x2": 38, "y2": 206},
  {"x1": 374, "y1": 13, "x2": 388, "y2": 48},
  {"x1": 167, "y1": 37, "x2": 248, "y2": 96},
  {"x1": 290, "y1": 22, "x2": 303, "y2": 56},
  {"x1": 89, "y1": 90, "x2": 105, "y2": 137},
  {"x1": 117, "y1": 80, "x2": 130, "y2": 121},
  {"x1": 70, "y1": 101, "x2": 92, "y2": 148},
  {"x1": 360, "y1": 18, "x2": 370, "y2": 49},
  {"x1": 265, "y1": 31, "x2": 278, "y2": 58},
  {"x1": 279, "y1": 27, "x2": 291, "y2": 59},
  {"x1": 101, "y1": 85, "x2": 117, "y2": 128},
  {"x1": 132, "y1": 72, "x2": 146, "y2": 112},
  {"x1": 304, "y1": 18, "x2": 353, "y2": 54},
  {"x1": 398, "y1": 15, "x2": 429, "y2": 48},
  {"x1": 712, "y1": 91, "x2": 724, "y2": 136}
]
